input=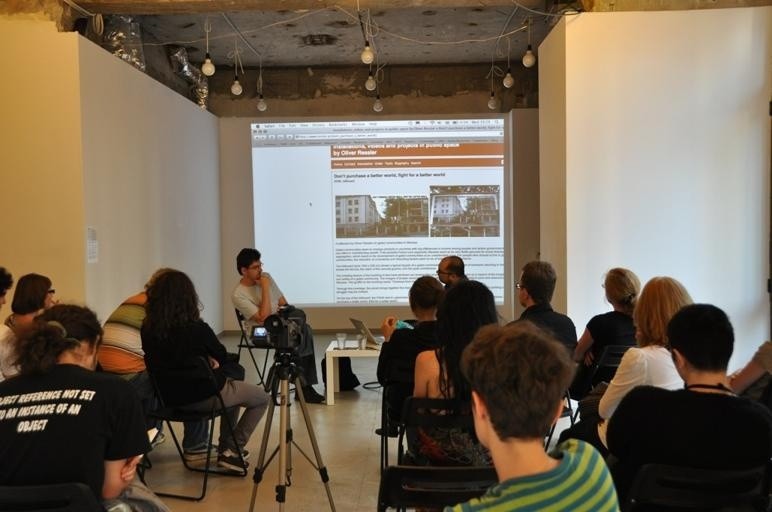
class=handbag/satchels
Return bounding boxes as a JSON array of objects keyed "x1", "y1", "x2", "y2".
[
  {"x1": 322, "y1": 353, "x2": 359, "y2": 390},
  {"x1": 578, "y1": 382, "x2": 610, "y2": 415}
]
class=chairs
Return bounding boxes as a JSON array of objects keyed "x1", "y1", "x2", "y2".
[
  {"x1": 235, "y1": 308, "x2": 295, "y2": 406},
  {"x1": 376, "y1": 359, "x2": 414, "y2": 477},
  {"x1": 543, "y1": 343, "x2": 578, "y2": 452},
  {"x1": 141, "y1": 344, "x2": 247, "y2": 501},
  {"x1": 377, "y1": 466, "x2": 499, "y2": 511},
  {"x1": 398, "y1": 397, "x2": 479, "y2": 465},
  {"x1": 571, "y1": 344, "x2": 638, "y2": 428}
]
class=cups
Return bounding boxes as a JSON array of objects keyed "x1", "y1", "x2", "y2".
[
  {"x1": 356, "y1": 334, "x2": 368, "y2": 350},
  {"x1": 336, "y1": 333, "x2": 347, "y2": 350}
]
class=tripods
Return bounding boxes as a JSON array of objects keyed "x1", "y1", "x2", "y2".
[{"x1": 249, "y1": 352, "x2": 335, "y2": 512}]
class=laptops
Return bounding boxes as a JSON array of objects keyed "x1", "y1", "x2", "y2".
[{"x1": 349, "y1": 318, "x2": 385, "y2": 345}]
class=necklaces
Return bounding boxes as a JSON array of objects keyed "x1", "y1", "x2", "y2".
[{"x1": 687, "y1": 382, "x2": 738, "y2": 395}]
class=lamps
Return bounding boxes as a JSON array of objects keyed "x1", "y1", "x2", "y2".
[
  {"x1": 522, "y1": 17, "x2": 536, "y2": 68},
  {"x1": 257, "y1": 55, "x2": 267, "y2": 112},
  {"x1": 361, "y1": 8, "x2": 374, "y2": 64},
  {"x1": 373, "y1": 55, "x2": 383, "y2": 112},
  {"x1": 503, "y1": 38, "x2": 514, "y2": 88},
  {"x1": 488, "y1": 56, "x2": 497, "y2": 109},
  {"x1": 201, "y1": 15, "x2": 215, "y2": 76},
  {"x1": 365, "y1": 64, "x2": 376, "y2": 91},
  {"x1": 231, "y1": 34, "x2": 242, "y2": 95}
]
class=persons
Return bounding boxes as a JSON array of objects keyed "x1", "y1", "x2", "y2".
[
  {"x1": 606, "y1": 302, "x2": 771, "y2": 512},
  {"x1": 442, "y1": 318, "x2": 620, "y2": 512},
  {"x1": 557, "y1": 277, "x2": 695, "y2": 458},
  {"x1": 1, "y1": 267, "x2": 154, "y2": 512},
  {"x1": 377, "y1": 254, "x2": 511, "y2": 466},
  {"x1": 567, "y1": 266, "x2": 641, "y2": 400},
  {"x1": 503, "y1": 260, "x2": 578, "y2": 350},
  {"x1": 98, "y1": 268, "x2": 270, "y2": 471},
  {"x1": 232, "y1": 249, "x2": 325, "y2": 404}
]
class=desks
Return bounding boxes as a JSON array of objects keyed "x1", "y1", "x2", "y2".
[{"x1": 325, "y1": 340, "x2": 381, "y2": 406}]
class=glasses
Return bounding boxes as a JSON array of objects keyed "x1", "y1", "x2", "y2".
[{"x1": 516, "y1": 283, "x2": 528, "y2": 288}]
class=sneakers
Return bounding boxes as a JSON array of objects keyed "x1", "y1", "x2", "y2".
[
  {"x1": 184, "y1": 443, "x2": 218, "y2": 461},
  {"x1": 217, "y1": 449, "x2": 250, "y2": 472},
  {"x1": 147, "y1": 434, "x2": 166, "y2": 449},
  {"x1": 376, "y1": 426, "x2": 398, "y2": 437}
]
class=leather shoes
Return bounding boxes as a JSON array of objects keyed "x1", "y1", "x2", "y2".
[{"x1": 295, "y1": 385, "x2": 325, "y2": 403}]
